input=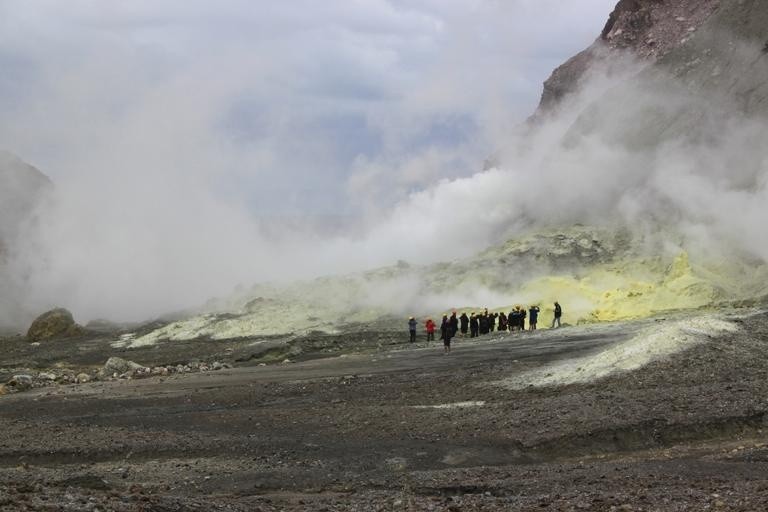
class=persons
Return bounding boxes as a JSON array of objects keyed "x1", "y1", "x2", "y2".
[{"x1": 408, "y1": 301, "x2": 562, "y2": 353}]
